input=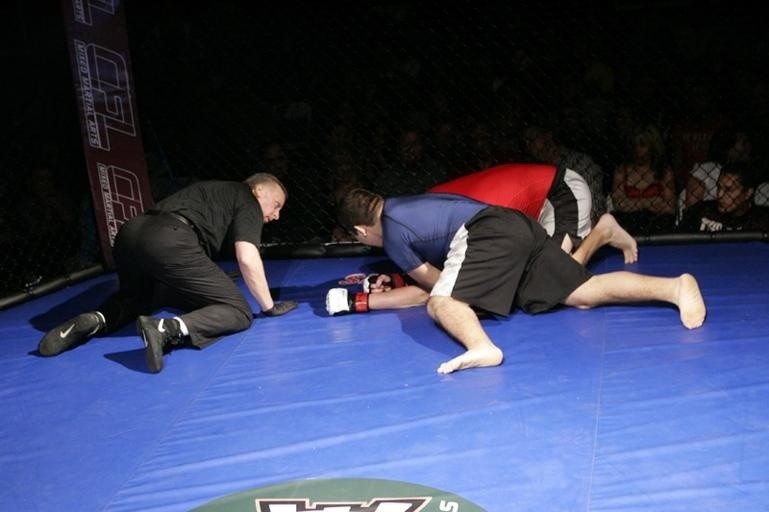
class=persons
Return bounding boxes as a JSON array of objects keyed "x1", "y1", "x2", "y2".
[
  {"x1": 1, "y1": 68, "x2": 768, "y2": 297},
  {"x1": 36, "y1": 174, "x2": 298, "y2": 374},
  {"x1": 323, "y1": 160, "x2": 640, "y2": 316},
  {"x1": 337, "y1": 185, "x2": 708, "y2": 375}
]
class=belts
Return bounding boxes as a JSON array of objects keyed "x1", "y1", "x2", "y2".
[{"x1": 168, "y1": 213, "x2": 192, "y2": 227}]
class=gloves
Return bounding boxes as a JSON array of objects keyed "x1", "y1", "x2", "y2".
[
  {"x1": 226, "y1": 271, "x2": 240, "y2": 280},
  {"x1": 261, "y1": 302, "x2": 297, "y2": 316}
]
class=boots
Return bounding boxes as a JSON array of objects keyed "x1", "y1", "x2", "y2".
[
  {"x1": 137, "y1": 316, "x2": 183, "y2": 373},
  {"x1": 39, "y1": 313, "x2": 104, "y2": 355}
]
